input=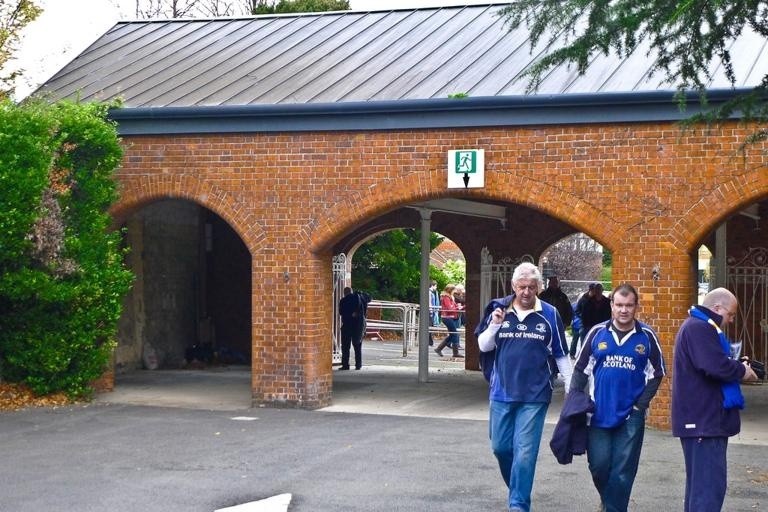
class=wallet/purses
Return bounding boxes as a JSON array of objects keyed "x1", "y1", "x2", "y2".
[{"x1": 741, "y1": 358, "x2": 765, "y2": 380}]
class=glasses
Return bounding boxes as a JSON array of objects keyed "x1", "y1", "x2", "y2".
[{"x1": 721, "y1": 305, "x2": 736, "y2": 318}]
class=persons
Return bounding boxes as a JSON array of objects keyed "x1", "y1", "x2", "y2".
[
  {"x1": 576, "y1": 283, "x2": 598, "y2": 318},
  {"x1": 338, "y1": 286, "x2": 373, "y2": 370},
  {"x1": 451, "y1": 284, "x2": 464, "y2": 330},
  {"x1": 536, "y1": 276, "x2": 573, "y2": 331},
  {"x1": 472, "y1": 261, "x2": 574, "y2": 512},
  {"x1": 580, "y1": 283, "x2": 611, "y2": 328},
  {"x1": 562, "y1": 280, "x2": 667, "y2": 512},
  {"x1": 434, "y1": 283, "x2": 464, "y2": 358},
  {"x1": 670, "y1": 286, "x2": 760, "y2": 512},
  {"x1": 569, "y1": 291, "x2": 589, "y2": 361},
  {"x1": 429, "y1": 279, "x2": 439, "y2": 347}
]
